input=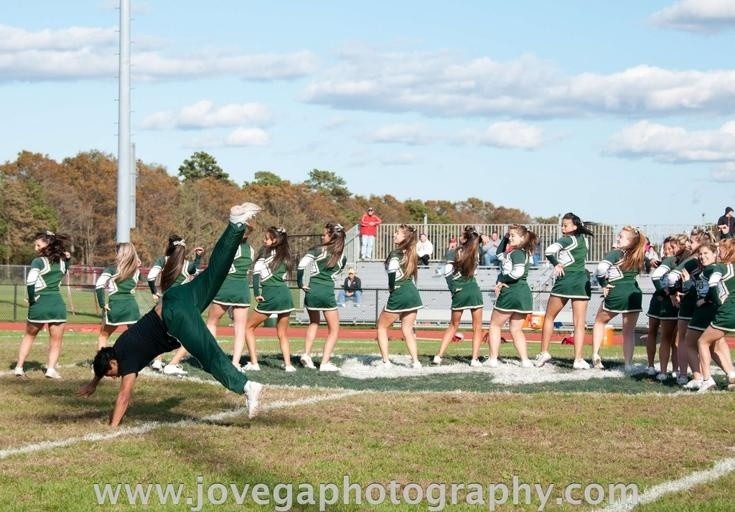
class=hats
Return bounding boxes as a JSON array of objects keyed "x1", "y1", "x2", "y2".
[{"x1": 348, "y1": 269, "x2": 354, "y2": 275}]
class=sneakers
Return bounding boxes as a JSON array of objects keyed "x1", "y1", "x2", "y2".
[
  {"x1": 244, "y1": 380, "x2": 264, "y2": 419},
  {"x1": 624, "y1": 363, "x2": 735, "y2": 393},
  {"x1": 229, "y1": 201, "x2": 261, "y2": 224},
  {"x1": 13, "y1": 367, "x2": 63, "y2": 379}
]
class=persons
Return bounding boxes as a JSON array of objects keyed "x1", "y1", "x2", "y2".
[
  {"x1": 146, "y1": 233, "x2": 204, "y2": 376},
  {"x1": 91, "y1": 242, "x2": 147, "y2": 374},
  {"x1": 204, "y1": 221, "x2": 258, "y2": 374},
  {"x1": 429, "y1": 205, "x2": 735, "y2": 393},
  {"x1": 294, "y1": 220, "x2": 348, "y2": 373},
  {"x1": 71, "y1": 201, "x2": 264, "y2": 427},
  {"x1": 415, "y1": 233, "x2": 435, "y2": 269},
  {"x1": 369, "y1": 224, "x2": 425, "y2": 369},
  {"x1": 239, "y1": 225, "x2": 297, "y2": 373},
  {"x1": 336, "y1": 268, "x2": 363, "y2": 306},
  {"x1": 359, "y1": 207, "x2": 383, "y2": 262},
  {"x1": 13, "y1": 231, "x2": 75, "y2": 379}
]
